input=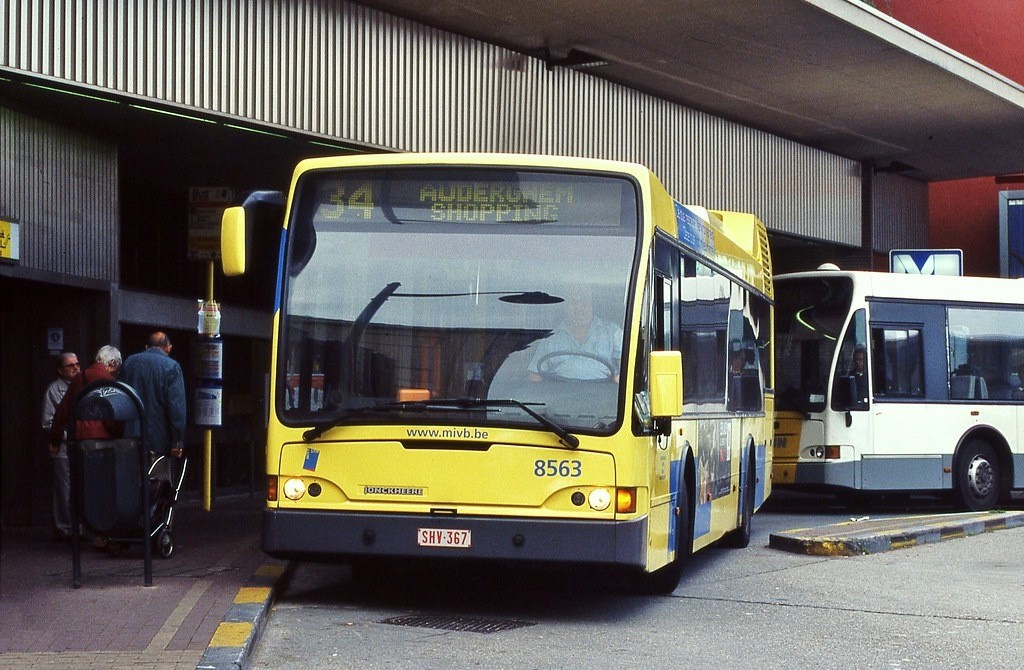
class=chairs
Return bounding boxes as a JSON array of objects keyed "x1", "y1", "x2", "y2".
[
  {"x1": 949, "y1": 375, "x2": 976, "y2": 399},
  {"x1": 974, "y1": 376, "x2": 989, "y2": 399}
]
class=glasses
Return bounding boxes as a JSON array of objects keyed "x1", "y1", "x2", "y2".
[{"x1": 854, "y1": 358, "x2": 864, "y2": 362}]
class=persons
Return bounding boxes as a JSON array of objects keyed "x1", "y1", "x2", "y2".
[
  {"x1": 528, "y1": 282, "x2": 623, "y2": 384},
  {"x1": 43, "y1": 332, "x2": 186, "y2": 550},
  {"x1": 956, "y1": 364, "x2": 982, "y2": 399},
  {"x1": 1015, "y1": 364, "x2": 1024, "y2": 400},
  {"x1": 848, "y1": 348, "x2": 868, "y2": 399},
  {"x1": 726, "y1": 338, "x2": 745, "y2": 415}
]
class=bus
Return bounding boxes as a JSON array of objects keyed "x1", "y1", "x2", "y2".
[
  {"x1": 730, "y1": 263, "x2": 1023, "y2": 514},
  {"x1": 221, "y1": 152, "x2": 776, "y2": 594}
]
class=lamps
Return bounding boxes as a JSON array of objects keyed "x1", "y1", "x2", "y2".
[{"x1": 544, "y1": 48, "x2": 610, "y2": 71}]
